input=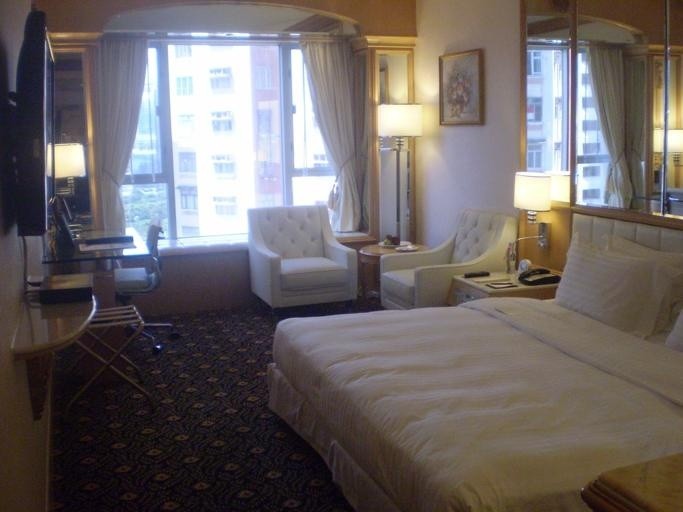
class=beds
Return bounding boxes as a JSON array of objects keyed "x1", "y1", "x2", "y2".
[{"x1": 267, "y1": 221, "x2": 683, "y2": 511}]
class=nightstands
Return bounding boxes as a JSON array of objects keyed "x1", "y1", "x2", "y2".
[{"x1": 447, "y1": 267, "x2": 562, "y2": 303}]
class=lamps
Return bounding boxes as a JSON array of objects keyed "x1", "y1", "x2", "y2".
[
  {"x1": 665, "y1": 128, "x2": 682, "y2": 167},
  {"x1": 378, "y1": 103, "x2": 423, "y2": 239},
  {"x1": 513, "y1": 171, "x2": 551, "y2": 224},
  {"x1": 652, "y1": 127, "x2": 664, "y2": 164}
]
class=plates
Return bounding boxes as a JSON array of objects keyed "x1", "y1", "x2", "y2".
[
  {"x1": 378, "y1": 241, "x2": 411, "y2": 247},
  {"x1": 393, "y1": 245, "x2": 419, "y2": 251}
]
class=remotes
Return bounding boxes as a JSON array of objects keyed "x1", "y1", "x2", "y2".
[{"x1": 463, "y1": 270, "x2": 490, "y2": 278}]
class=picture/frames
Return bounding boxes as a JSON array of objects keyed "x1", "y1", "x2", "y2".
[{"x1": 438, "y1": 48, "x2": 484, "y2": 126}]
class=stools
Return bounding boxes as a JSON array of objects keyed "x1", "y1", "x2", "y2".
[
  {"x1": 61, "y1": 306, "x2": 155, "y2": 422},
  {"x1": 46, "y1": 143, "x2": 86, "y2": 198}
]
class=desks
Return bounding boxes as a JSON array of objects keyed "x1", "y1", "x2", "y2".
[
  {"x1": 42, "y1": 225, "x2": 152, "y2": 266},
  {"x1": 360, "y1": 241, "x2": 429, "y2": 308},
  {"x1": 581, "y1": 454, "x2": 683, "y2": 512}
]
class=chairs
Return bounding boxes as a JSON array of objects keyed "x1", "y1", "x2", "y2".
[{"x1": 113, "y1": 224, "x2": 179, "y2": 353}]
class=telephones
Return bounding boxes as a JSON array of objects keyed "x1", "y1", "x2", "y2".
[{"x1": 518, "y1": 268, "x2": 561, "y2": 285}]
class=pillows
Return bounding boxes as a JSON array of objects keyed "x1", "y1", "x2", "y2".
[
  {"x1": 555, "y1": 234, "x2": 673, "y2": 339},
  {"x1": 608, "y1": 234, "x2": 682, "y2": 334},
  {"x1": 665, "y1": 311, "x2": 683, "y2": 351}
]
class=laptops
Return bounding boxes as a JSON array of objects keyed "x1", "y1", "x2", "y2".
[{"x1": 82, "y1": 227, "x2": 134, "y2": 246}]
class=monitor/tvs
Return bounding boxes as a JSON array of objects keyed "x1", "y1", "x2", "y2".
[{"x1": 0, "y1": 10, "x2": 57, "y2": 238}]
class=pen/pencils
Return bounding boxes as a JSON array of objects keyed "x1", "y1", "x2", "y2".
[{"x1": 492, "y1": 283, "x2": 512, "y2": 285}]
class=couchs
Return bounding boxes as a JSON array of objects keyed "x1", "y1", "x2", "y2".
[
  {"x1": 380, "y1": 209, "x2": 518, "y2": 310},
  {"x1": 247, "y1": 205, "x2": 359, "y2": 309}
]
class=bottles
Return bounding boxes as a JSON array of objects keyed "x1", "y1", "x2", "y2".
[{"x1": 506, "y1": 242, "x2": 517, "y2": 274}]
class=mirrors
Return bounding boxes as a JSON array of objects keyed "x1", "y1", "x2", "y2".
[
  {"x1": 52, "y1": 45, "x2": 104, "y2": 261},
  {"x1": 372, "y1": 49, "x2": 417, "y2": 245},
  {"x1": 520, "y1": 0, "x2": 683, "y2": 228}
]
